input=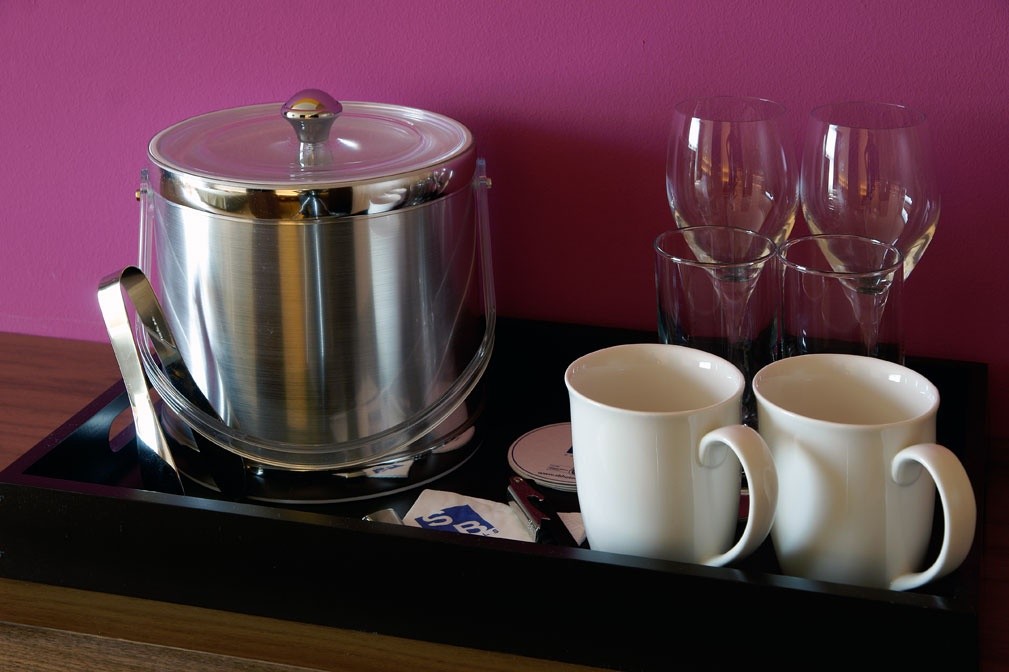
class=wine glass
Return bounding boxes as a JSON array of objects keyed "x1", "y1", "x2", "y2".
[
  {"x1": 801, "y1": 98, "x2": 942, "y2": 356},
  {"x1": 666, "y1": 94, "x2": 801, "y2": 373}
]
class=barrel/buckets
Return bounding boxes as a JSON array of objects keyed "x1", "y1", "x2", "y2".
[{"x1": 133, "y1": 86, "x2": 497, "y2": 504}]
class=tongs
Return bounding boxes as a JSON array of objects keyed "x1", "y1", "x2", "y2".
[{"x1": 96, "y1": 263, "x2": 251, "y2": 503}]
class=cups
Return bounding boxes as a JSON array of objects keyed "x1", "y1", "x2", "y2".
[
  {"x1": 653, "y1": 225, "x2": 778, "y2": 425},
  {"x1": 563, "y1": 342, "x2": 778, "y2": 570},
  {"x1": 751, "y1": 354, "x2": 977, "y2": 593},
  {"x1": 777, "y1": 233, "x2": 905, "y2": 359}
]
